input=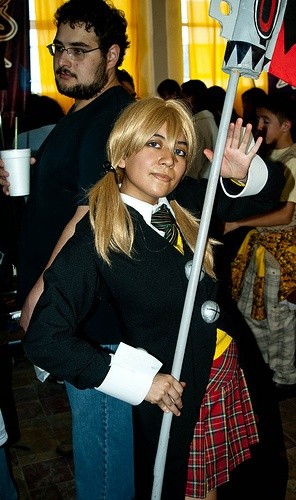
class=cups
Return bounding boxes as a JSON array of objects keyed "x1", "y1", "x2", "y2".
[{"x1": 0, "y1": 148, "x2": 31, "y2": 196}]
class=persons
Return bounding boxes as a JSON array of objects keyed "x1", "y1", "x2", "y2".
[
  {"x1": 1, "y1": 68, "x2": 295, "y2": 365},
  {"x1": 21, "y1": 96, "x2": 289, "y2": 500},
  {"x1": 216, "y1": 91, "x2": 296, "y2": 388},
  {"x1": 0, "y1": 1, "x2": 136, "y2": 500}
]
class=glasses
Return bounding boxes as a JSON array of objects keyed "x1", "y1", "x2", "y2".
[{"x1": 47, "y1": 42, "x2": 114, "y2": 61}]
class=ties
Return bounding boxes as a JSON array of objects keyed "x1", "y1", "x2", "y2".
[{"x1": 151, "y1": 204, "x2": 179, "y2": 247}]
